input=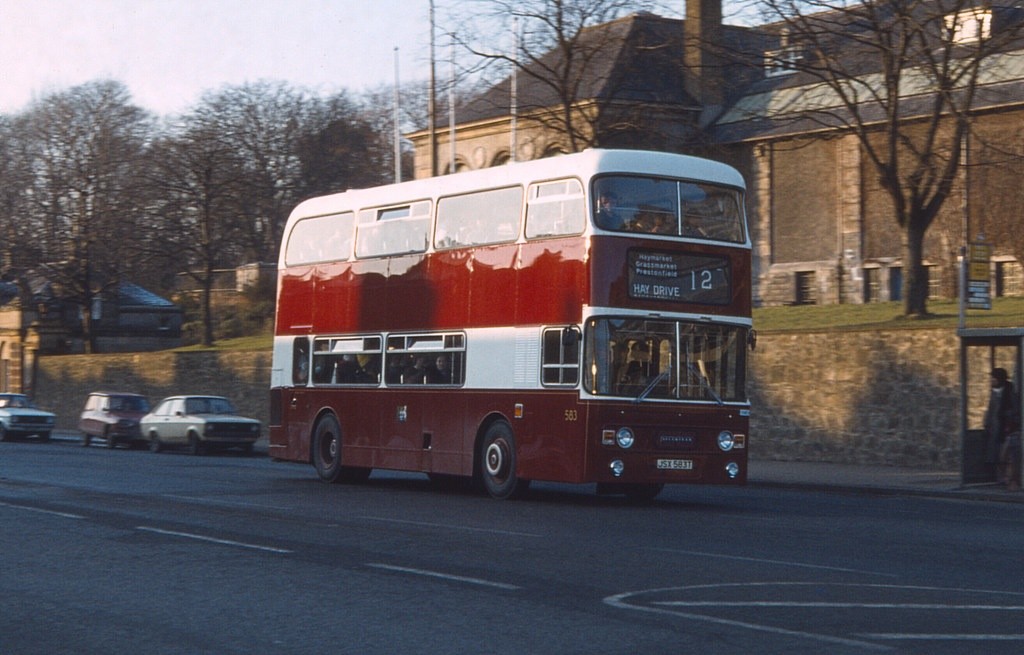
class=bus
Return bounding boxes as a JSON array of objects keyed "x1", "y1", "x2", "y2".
[{"x1": 267, "y1": 146, "x2": 757, "y2": 500}]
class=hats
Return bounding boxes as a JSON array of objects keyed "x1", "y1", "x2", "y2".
[
  {"x1": 356, "y1": 353, "x2": 376, "y2": 367},
  {"x1": 987, "y1": 368, "x2": 1012, "y2": 380}
]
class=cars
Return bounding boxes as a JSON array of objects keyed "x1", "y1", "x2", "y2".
[
  {"x1": 0, "y1": 393, "x2": 56, "y2": 441},
  {"x1": 139, "y1": 394, "x2": 262, "y2": 453}
]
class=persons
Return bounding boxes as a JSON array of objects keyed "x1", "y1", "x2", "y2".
[
  {"x1": 295, "y1": 338, "x2": 458, "y2": 384},
  {"x1": 611, "y1": 341, "x2": 659, "y2": 397},
  {"x1": 595, "y1": 195, "x2": 697, "y2": 237},
  {"x1": 984, "y1": 368, "x2": 1016, "y2": 480}
]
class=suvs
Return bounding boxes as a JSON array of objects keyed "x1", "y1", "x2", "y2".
[{"x1": 80, "y1": 391, "x2": 150, "y2": 448}]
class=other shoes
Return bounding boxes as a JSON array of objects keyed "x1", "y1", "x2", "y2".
[{"x1": 1008, "y1": 481, "x2": 1021, "y2": 492}]
